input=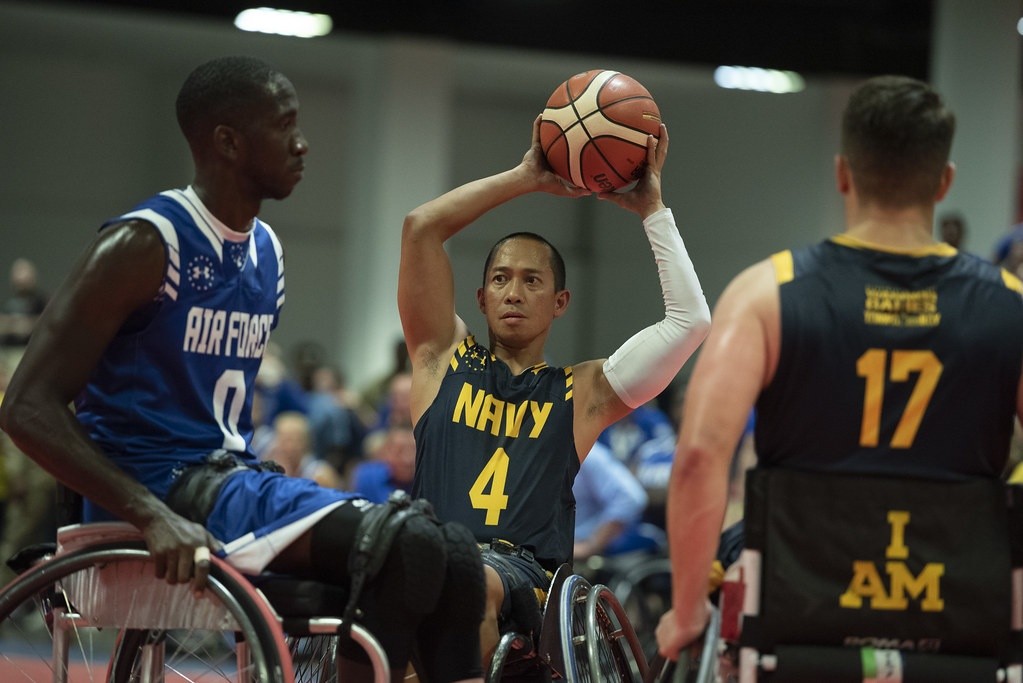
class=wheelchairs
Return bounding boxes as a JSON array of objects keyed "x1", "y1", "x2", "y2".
[
  {"x1": 279, "y1": 563, "x2": 655, "y2": 683},
  {"x1": 0, "y1": 476, "x2": 387, "y2": 683},
  {"x1": 669, "y1": 469, "x2": 1023, "y2": 683}
]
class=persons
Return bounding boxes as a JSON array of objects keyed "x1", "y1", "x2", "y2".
[
  {"x1": 657, "y1": 77, "x2": 1023, "y2": 683},
  {"x1": 398, "y1": 112, "x2": 711, "y2": 683},
  {"x1": 0, "y1": 56, "x2": 485, "y2": 683},
  {"x1": 573, "y1": 405, "x2": 677, "y2": 580},
  {"x1": 1, "y1": 258, "x2": 56, "y2": 558},
  {"x1": 247, "y1": 333, "x2": 417, "y2": 501}
]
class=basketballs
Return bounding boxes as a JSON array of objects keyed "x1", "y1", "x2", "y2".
[{"x1": 541, "y1": 69, "x2": 662, "y2": 193}]
